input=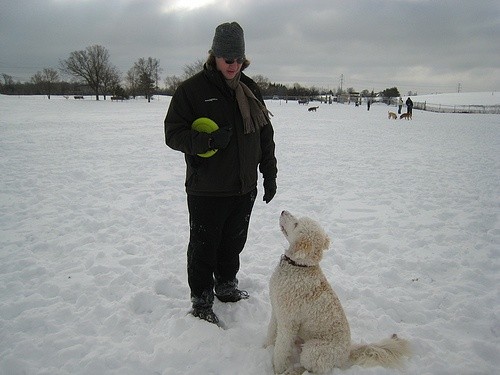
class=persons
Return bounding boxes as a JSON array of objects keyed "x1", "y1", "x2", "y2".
[
  {"x1": 406, "y1": 97, "x2": 413, "y2": 117},
  {"x1": 366, "y1": 100, "x2": 371, "y2": 110},
  {"x1": 397, "y1": 97, "x2": 403, "y2": 114},
  {"x1": 163, "y1": 21, "x2": 277, "y2": 327}
]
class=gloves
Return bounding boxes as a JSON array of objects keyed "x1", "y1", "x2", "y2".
[
  {"x1": 209, "y1": 128, "x2": 231, "y2": 149},
  {"x1": 263, "y1": 178, "x2": 277, "y2": 204}
]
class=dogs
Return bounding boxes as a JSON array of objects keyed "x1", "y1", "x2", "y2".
[
  {"x1": 261, "y1": 209, "x2": 411, "y2": 374},
  {"x1": 308, "y1": 106, "x2": 319, "y2": 112},
  {"x1": 387, "y1": 110, "x2": 413, "y2": 121}
]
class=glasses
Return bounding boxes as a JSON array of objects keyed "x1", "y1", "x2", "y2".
[{"x1": 223, "y1": 58, "x2": 245, "y2": 64}]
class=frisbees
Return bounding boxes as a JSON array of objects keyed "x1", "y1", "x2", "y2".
[{"x1": 191, "y1": 117, "x2": 219, "y2": 157}]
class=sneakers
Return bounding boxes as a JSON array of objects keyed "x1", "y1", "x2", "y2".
[
  {"x1": 215, "y1": 289, "x2": 242, "y2": 302},
  {"x1": 193, "y1": 308, "x2": 220, "y2": 324}
]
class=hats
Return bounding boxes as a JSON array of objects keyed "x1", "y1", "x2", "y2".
[{"x1": 212, "y1": 22, "x2": 245, "y2": 58}]
